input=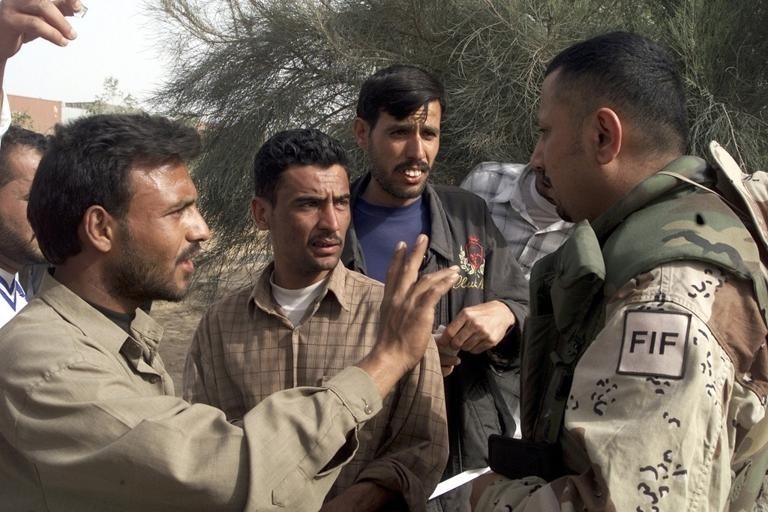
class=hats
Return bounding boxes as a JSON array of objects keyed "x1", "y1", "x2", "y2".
[{"x1": 708, "y1": 134, "x2": 768, "y2": 253}]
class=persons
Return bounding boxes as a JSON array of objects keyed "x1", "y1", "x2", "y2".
[
  {"x1": 182, "y1": 131, "x2": 450, "y2": 512},
  {"x1": 339, "y1": 60, "x2": 533, "y2": 512},
  {"x1": 459, "y1": 157, "x2": 577, "y2": 438},
  {"x1": 0, "y1": 113, "x2": 457, "y2": 512},
  {"x1": 0, "y1": 1, "x2": 83, "y2": 333},
  {"x1": 442, "y1": 32, "x2": 768, "y2": 512}
]
